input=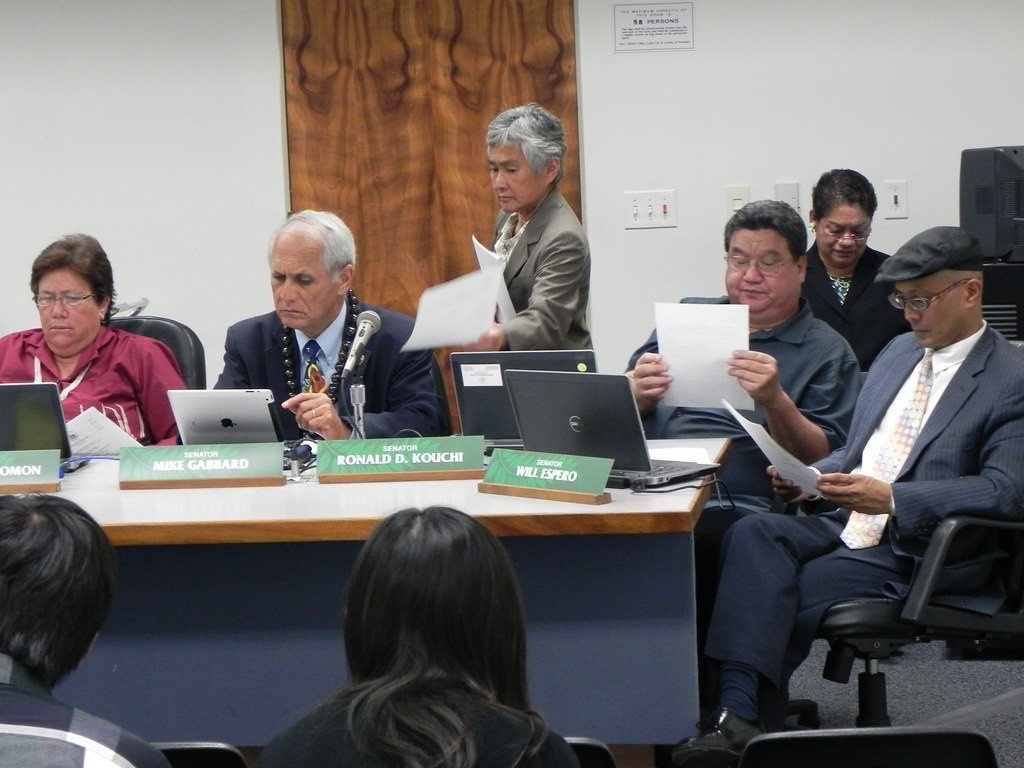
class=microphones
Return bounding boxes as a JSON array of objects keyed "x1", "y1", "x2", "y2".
[{"x1": 342, "y1": 311, "x2": 382, "y2": 380}]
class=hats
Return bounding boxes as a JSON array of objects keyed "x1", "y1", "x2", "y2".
[{"x1": 876, "y1": 226, "x2": 983, "y2": 282}]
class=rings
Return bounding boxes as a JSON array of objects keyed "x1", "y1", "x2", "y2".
[{"x1": 312, "y1": 409, "x2": 316, "y2": 418}]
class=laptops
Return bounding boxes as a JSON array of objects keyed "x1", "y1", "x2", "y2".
[
  {"x1": 0, "y1": 383, "x2": 93, "y2": 472},
  {"x1": 448, "y1": 351, "x2": 721, "y2": 488},
  {"x1": 166, "y1": 389, "x2": 302, "y2": 449}
]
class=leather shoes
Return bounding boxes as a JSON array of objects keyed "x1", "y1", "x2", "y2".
[{"x1": 672, "y1": 707, "x2": 767, "y2": 768}]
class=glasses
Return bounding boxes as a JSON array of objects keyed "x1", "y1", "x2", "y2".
[
  {"x1": 890, "y1": 276, "x2": 970, "y2": 311},
  {"x1": 820, "y1": 218, "x2": 873, "y2": 242},
  {"x1": 31, "y1": 290, "x2": 98, "y2": 309},
  {"x1": 726, "y1": 255, "x2": 802, "y2": 274}
]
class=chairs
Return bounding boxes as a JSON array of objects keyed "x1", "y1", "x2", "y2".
[
  {"x1": 815, "y1": 511, "x2": 1024, "y2": 728},
  {"x1": 739, "y1": 723, "x2": 998, "y2": 768},
  {"x1": 109, "y1": 313, "x2": 207, "y2": 401}
]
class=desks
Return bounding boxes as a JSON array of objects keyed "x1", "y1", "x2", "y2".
[{"x1": 0, "y1": 432, "x2": 735, "y2": 744}]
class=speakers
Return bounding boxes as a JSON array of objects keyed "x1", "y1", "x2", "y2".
[{"x1": 959, "y1": 145, "x2": 1024, "y2": 263}]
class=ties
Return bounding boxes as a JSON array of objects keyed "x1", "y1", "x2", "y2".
[
  {"x1": 302, "y1": 342, "x2": 322, "y2": 395},
  {"x1": 840, "y1": 356, "x2": 933, "y2": 548}
]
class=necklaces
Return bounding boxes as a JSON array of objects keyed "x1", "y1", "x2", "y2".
[{"x1": 281, "y1": 289, "x2": 359, "y2": 404}]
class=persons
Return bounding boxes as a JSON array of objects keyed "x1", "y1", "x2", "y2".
[
  {"x1": 0, "y1": 494, "x2": 171, "y2": 768},
  {"x1": 801, "y1": 169, "x2": 914, "y2": 373},
  {"x1": 214, "y1": 209, "x2": 440, "y2": 441},
  {"x1": 260, "y1": 506, "x2": 580, "y2": 768},
  {"x1": 672, "y1": 226, "x2": 1024, "y2": 768},
  {"x1": 0, "y1": 233, "x2": 188, "y2": 447},
  {"x1": 462, "y1": 102, "x2": 592, "y2": 352},
  {"x1": 625, "y1": 199, "x2": 860, "y2": 515}
]
